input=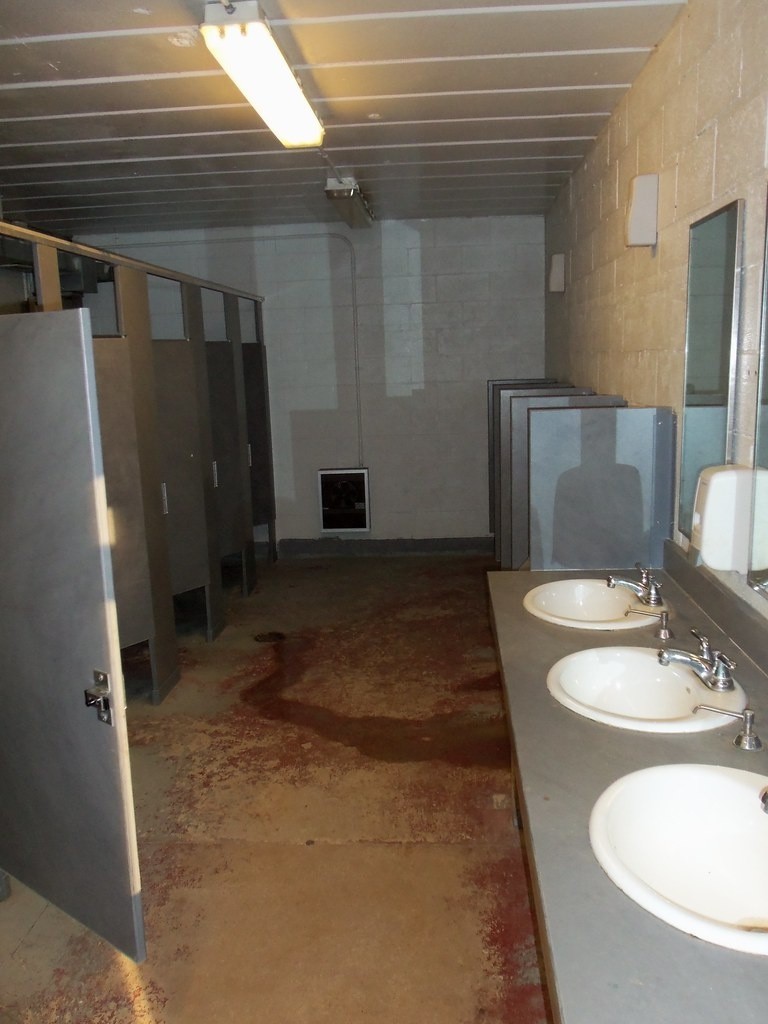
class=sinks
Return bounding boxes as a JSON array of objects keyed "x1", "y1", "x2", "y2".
[
  {"x1": 590, "y1": 764, "x2": 768, "y2": 956},
  {"x1": 547, "y1": 645, "x2": 746, "y2": 734},
  {"x1": 523, "y1": 577, "x2": 669, "y2": 630}
]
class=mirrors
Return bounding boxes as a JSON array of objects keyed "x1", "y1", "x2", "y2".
[{"x1": 676, "y1": 183, "x2": 768, "y2": 598}]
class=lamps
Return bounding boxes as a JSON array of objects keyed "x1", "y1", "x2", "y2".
[
  {"x1": 324, "y1": 177, "x2": 374, "y2": 229},
  {"x1": 198, "y1": 0, "x2": 326, "y2": 148}
]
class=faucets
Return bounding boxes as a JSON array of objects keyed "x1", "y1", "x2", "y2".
[
  {"x1": 659, "y1": 629, "x2": 735, "y2": 692},
  {"x1": 605, "y1": 563, "x2": 663, "y2": 608}
]
